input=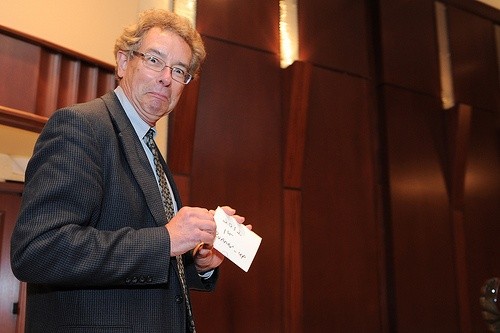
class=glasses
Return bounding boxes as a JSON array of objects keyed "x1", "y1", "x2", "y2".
[{"x1": 120, "y1": 49, "x2": 194, "y2": 85}]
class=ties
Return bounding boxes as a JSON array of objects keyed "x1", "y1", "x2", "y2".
[{"x1": 143, "y1": 130, "x2": 196, "y2": 333}]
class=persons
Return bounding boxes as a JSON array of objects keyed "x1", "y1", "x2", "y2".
[{"x1": 9, "y1": 8, "x2": 252, "y2": 333}]
